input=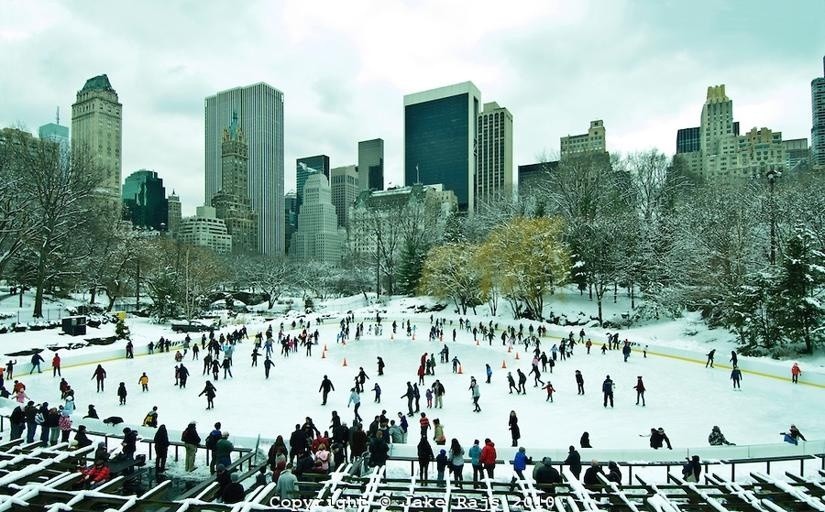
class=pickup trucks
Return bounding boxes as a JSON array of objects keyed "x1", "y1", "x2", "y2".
[{"x1": 171, "y1": 317, "x2": 213, "y2": 333}]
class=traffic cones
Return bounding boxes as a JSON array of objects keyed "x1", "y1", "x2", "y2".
[
  {"x1": 391, "y1": 333, "x2": 394, "y2": 340},
  {"x1": 412, "y1": 334, "x2": 415, "y2": 340},
  {"x1": 341, "y1": 358, "x2": 347, "y2": 366},
  {"x1": 324, "y1": 345, "x2": 327, "y2": 351},
  {"x1": 502, "y1": 346, "x2": 520, "y2": 368},
  {"x1": 322, "y1": 351, "x2": 326, "y2": 359},
  {"x1": 457, "y1": 365, "x2": 463, "y2": 374},
  {"x1": 476, "y1": 339, "x2": 480, "y2": 345},
  {"x1": 342, "y1": 339, "x2": 346, "y2": 345},
  {"x1": 439, "y1": 336, "x2": 443, "y2": 341}
]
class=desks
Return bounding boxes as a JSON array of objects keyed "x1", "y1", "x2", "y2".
[{"x1": 94, "y1": 458, "x2": 141, "y2": 489}]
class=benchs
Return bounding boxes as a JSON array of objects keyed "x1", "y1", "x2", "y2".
[{"x1": 120, "y1": 468, "x2": 148, "y2": 490}]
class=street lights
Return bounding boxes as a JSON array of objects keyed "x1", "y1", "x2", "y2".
[{"x1": 755, "y1": 166, "x2": 783, "y2": 264}]
